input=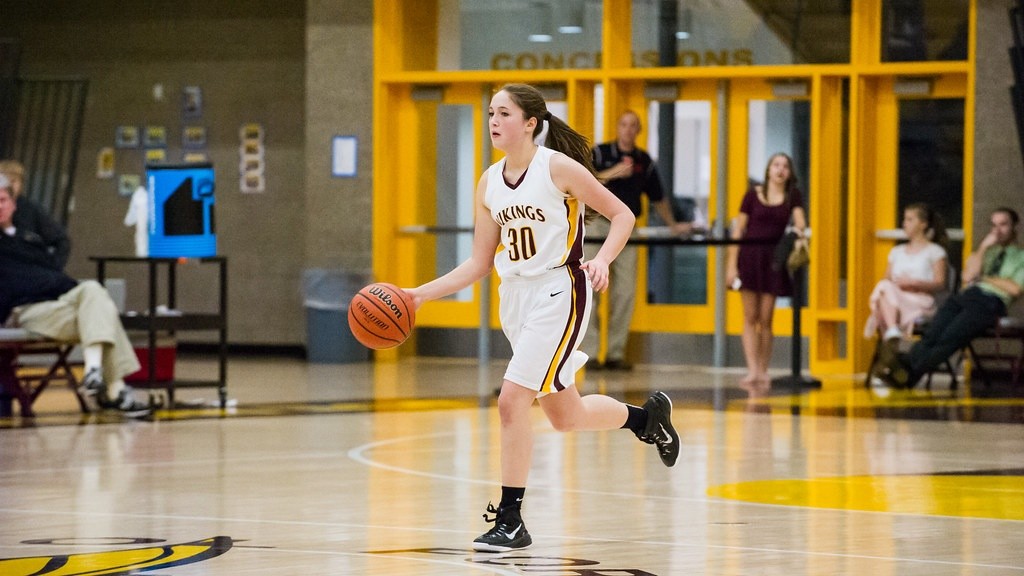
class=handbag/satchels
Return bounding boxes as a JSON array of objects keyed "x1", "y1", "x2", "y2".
[{"x1": 788, "y1": 247, "x2": 809, "y2": 270}]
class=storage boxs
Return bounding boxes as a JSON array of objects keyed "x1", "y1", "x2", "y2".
[
  {"x1": 146, "y1": 163, "x2": 217, "y2": 255},
  {"x1": 125, "y1": 339, "x2": 178, "y2": 382}
]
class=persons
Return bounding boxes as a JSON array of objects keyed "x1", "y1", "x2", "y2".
[
  {"x1": 397, "y1": 83, "x2": 679, "y2": 560},
  {"x1": 0, "y1": 153, "x2": 165, "y2": 423},
  {"x1": 862, "y1": 204, "x2": 1024, "y2": 391},
  {"x1": 724, "y1": 152, "x2": 810, "y2": 391},
  {"x1": 583, "y1": 109, "x2": 695, "y2": 373}
]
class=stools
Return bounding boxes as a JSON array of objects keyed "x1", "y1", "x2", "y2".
[{"x1": 0, "y1": 326, "x2": 90, "y2": 418}]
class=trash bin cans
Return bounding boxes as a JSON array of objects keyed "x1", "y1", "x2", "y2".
[{"x1": 299, "y1": 268, "x2": 371, "y2": 362}]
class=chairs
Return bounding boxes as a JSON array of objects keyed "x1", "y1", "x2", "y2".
[
  {"x1": 948, "y1": 290, "x2": 1024, "y2": 392},
  {"x1": 863, "y1": 258, "x2": 969, "y2": 392}
]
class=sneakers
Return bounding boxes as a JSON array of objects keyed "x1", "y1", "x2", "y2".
[
  {"x1": 77, "y1": 367, "x2": 106, "y2": 411},
  {"x1": 97, "y1": 384, "x2": 150, "y2": 418},
  {"x1": 472, "y1": 501, "x2": 533, "y2": 551},
  {"x1": 636, "y1": 390, "x2": 680, "y2": 467}
]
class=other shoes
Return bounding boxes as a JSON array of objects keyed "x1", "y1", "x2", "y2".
[
  {"x1": 878, "y1": 352, "x2": 921, "y2": 389},
  {"x1": 872, "y1": 377, "x2": 884, "y2": 385},
  {"x1": 585, "y1": 358, "x2": 602, "y2": 371},
  {"x1": 604, "y1": 359, "x2": 631, "y2": 371},
  {"x1": 884, "y1": 326, "x2": 901, "y2": 343}
]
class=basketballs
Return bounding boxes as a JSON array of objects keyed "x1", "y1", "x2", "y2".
[{"x1": 346, "y1": 281, "x2": 418, "y2": 350}]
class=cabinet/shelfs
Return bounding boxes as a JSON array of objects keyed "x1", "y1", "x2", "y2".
[{"x1": 88, "y1": 256, "x2": 229, "y2": 411}]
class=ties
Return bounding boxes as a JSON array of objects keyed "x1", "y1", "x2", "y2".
[{"x1": 987, "y1": 251, "x2": 1005, "y2": 275}]
